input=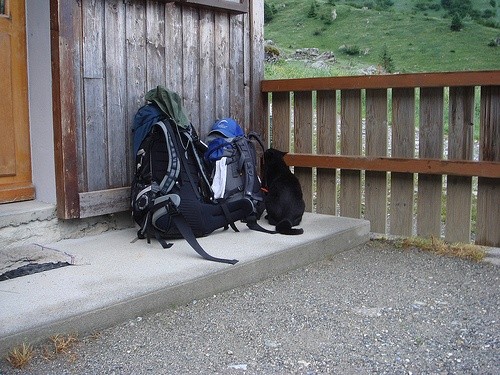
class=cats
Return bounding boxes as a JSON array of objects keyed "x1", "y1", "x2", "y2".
[{"x1": 261, "y1": 148, "x2": 306, "y2": 236}]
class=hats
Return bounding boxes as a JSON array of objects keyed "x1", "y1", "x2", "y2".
[
  {"x1": 207, "y1": 118, "x2": 244, "y2": 137},
  {"x1": 144, "y1": 85, "x2": 190, "y2": 130}
]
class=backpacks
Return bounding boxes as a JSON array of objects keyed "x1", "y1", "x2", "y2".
[
  {"x1": 204, "y1": 131, "x2": 266, "y2": 219},
  {"x1": 131, "y1": 104, "x2": 253, "y2": 241}
]
